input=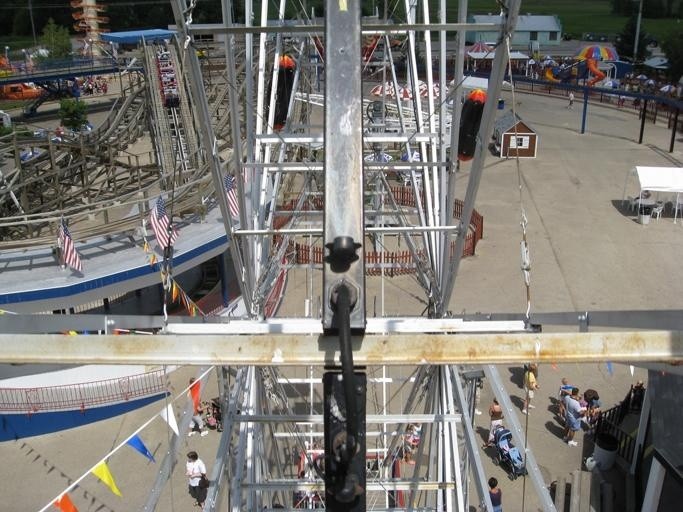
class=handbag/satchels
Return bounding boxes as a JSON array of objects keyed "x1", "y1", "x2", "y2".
[{"x1": 199, "y1": 479, "x2": 211, "y2": 490}]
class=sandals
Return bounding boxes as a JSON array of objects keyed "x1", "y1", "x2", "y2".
[{"x1": 193, "y1": 501, "x2": 205, "y2": 511}]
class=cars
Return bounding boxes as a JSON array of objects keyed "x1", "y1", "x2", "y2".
[{"x1": 196, "y1": 46, "x2": 216, "y2": 59}]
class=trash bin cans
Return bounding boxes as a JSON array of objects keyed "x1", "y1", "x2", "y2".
[
  {"x1": 498, "y1": 99, "x2": 504, "y2": 109},
  {"x1": 639, "y1": 206, "x2": 651, "y2": 225}
]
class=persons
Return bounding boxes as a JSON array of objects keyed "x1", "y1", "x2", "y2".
[
  {"x1": 54, "y1": 127, "x2": 65, "y2": 137},
  {"x1": 400, "y1": 423, "x2": 415, "y2": 465},
  {"x1": 568, "y1": 91, "x2": 574, "y2": 109},
  {"x1": 412, "y1": 423, "x2": 423, "y2": 448},
  {"x1": 84, "y1": 75, "x2": 108, "y2": 97},
  {"x1": 520, "y1": 364, "x2": 540, "y2": 416},
  {"x1": 487, "y1": 476, "x2": 503, "y2": 512},
  {"x1": 486, "y1": 396, "x2": 504, "y2": 442},
  {"x1": 557, "y1": 377, "x2": 570, "y2": 417},
  {"x1": 562, "y1": 387, "x2": 588, "y2": 447},
  {"x1": 182, "y1": 450, "x2": 208, "y2": 510},
  {"x1": 185, "y1": 378, "x2": 208, "y2": 437}
]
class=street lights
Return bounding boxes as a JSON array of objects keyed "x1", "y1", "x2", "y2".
[
  {"x1": 4, "y1": 46, "x2": 9, "y2": 78},
  {"x1": 21, "y1": 49, "x2": 28, "y2": 75}
]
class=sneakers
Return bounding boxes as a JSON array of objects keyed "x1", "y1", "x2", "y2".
[
  {"x1": 475, "y1": 409, "x2": 483, "y2": 416},
  {"x1": 522, "y1": 403, "x2": 535, "y2": 415},
  {"x1": 188, "y1": 430, "x2": 208, "y2": 437},
  {"x1": 563, "y1": 436, "x2": 578, "y2": 446},
  {"x1": 407, "y1": 459, "x2": 415, "y2": 465}
]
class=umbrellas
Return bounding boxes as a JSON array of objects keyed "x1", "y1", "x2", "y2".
[
  {"x1": 604, "y1": 80, "x2": 619, "y2": 89},
  {"x1": 371, "y1": 79, "x2": 450, "y2": 103},
  {"x1": 659, "y1": 84, "x2": 676, "y2": 95},
  {"x1": 643, "y1": 79, "x2": 657, "y2": 87},
  {"x1": 635, "y1": 74, "x2": 649, "y2": 84}
]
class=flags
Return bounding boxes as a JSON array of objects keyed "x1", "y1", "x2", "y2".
[
  {"x1": 220, "y1": 171, "x2": 239, "y2": 220},
  {"x1": 55, "y1": 213, "x2": 83, "y2": 273},
  {"x1": 148, "y1": 196, "x2": 179, "y2": 251}
]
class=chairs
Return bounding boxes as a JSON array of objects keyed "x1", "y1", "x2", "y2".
[{"x1": 627, "y1": 195, "x2": 683, "y2": 221}]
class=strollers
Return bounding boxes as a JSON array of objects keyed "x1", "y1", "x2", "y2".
[{"x1": 493, "y1": 425, "x2": 528, "y2": 481}]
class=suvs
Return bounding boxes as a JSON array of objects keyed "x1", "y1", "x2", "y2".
[{"x1": 3, "y1": 84, "x2": 47, "y2": 101}]
